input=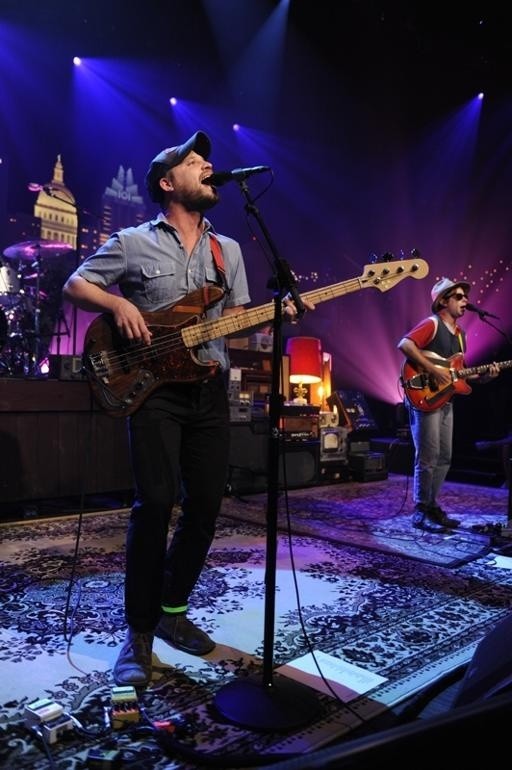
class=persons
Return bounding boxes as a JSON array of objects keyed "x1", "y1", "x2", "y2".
[
  {"x1": 64, "y1": 130, "x2": 317, "y2": 688},
  {"x1": 397, "y1": 277, "x2": 500, "y2": 533}
]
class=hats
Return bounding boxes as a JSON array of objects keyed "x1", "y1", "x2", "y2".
[
  {"x1": 431, "y1": 276, "x2": 471, "y2": 314},
  {"x1": 142, "y1": 129, "x2": 213, "y2": 204}
]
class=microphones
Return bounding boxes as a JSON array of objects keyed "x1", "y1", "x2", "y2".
[
  {"x1": 465, "y1": 302, "x2": 499, "y2": 322},
  {"x1": 210, "y1": 166, "x2": 271, "y2": 187}
]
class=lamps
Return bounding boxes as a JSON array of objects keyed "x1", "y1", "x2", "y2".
[{"x1": 285, "y1": 337, "x2": 323, "y2": 406}]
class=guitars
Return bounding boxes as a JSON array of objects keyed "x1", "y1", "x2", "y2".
[
  {"x1": 82, "y1": 248, "x2": 430, "y2": 419},
  {"x1": 397, "y1": 348, "x2": 510, "y2": 411}
]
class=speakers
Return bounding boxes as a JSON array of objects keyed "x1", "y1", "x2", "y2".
[
  {"x1": 227, "y1": 421, "x2": 271, "y2": 498},
  {"x1": 252, "y1": 694, "x2": 511, "y2": 770}
]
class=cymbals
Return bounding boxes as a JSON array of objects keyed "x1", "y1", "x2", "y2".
[{"x1": 3, "y1": 239, "x2": 73, "y2": 259}]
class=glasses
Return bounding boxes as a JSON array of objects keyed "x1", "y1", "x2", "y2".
[{"x1": 446, "y1": 292, "x2": 468, "y2": 301}]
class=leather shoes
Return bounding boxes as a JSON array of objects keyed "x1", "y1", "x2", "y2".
[
  {"x1": 411, "y1": 505, "x2": 446, "y2": 531},
  {"x1": 155, "y1": 611, "x2": 217, "y2": 655},
  {"x1": 433, "y1": 500, "x2": 460, "y2": 526},
  {"x1": 113, "y1": 630, "x2": 154, "y2": 689}
]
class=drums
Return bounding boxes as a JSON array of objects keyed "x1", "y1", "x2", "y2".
[
  {"x1": 24, "y1": 285, "x2": 50, "y2": 315},
  {"x1": 0, "y1": 266, "x2": 23, "y2": 307}
]
